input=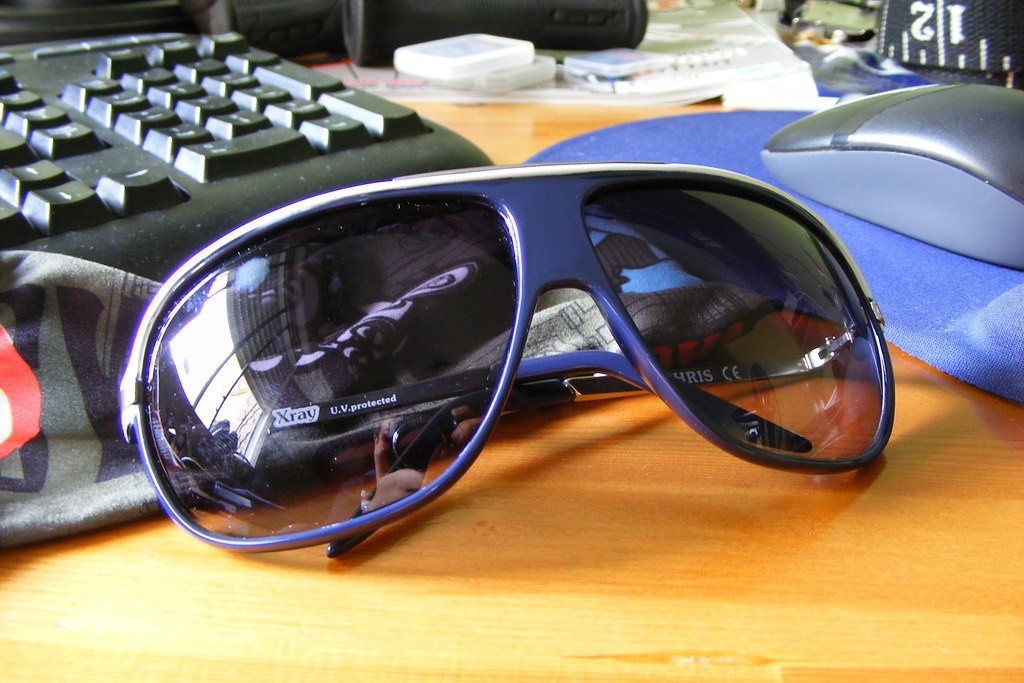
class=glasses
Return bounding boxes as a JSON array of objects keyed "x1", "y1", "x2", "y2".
[{"x1": 120, "y1": 162, "x2": 896, "y2": 558}]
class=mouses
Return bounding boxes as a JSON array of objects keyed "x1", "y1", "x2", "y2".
[{"x1": 761, "y1": 84, "x2": 1024, "y2": 273}]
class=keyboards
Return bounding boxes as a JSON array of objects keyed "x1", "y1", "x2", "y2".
[{"x1": 0, "y1": 27, "x2": 497, "y2": 284}]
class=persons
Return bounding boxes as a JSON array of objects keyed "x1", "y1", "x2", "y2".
[{"x1": 361, "y1": 403, "x2": 491, "y2": 509}]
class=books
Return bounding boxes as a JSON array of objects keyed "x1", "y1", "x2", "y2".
[{"x1": 312, "y1": 0, "x2": 806, "y2": 106}]
